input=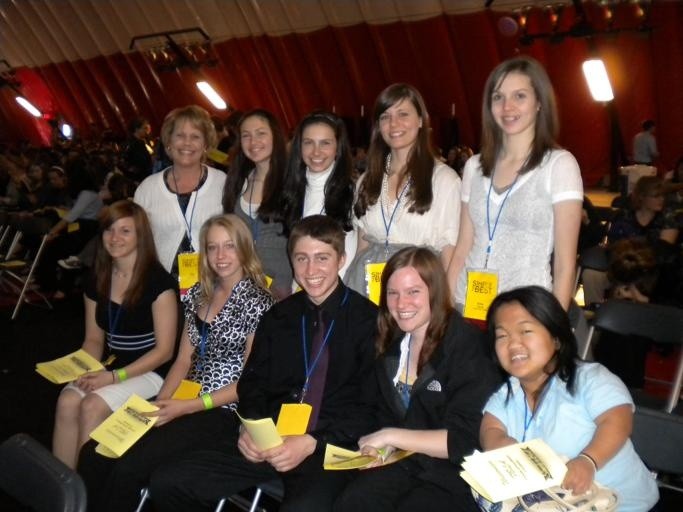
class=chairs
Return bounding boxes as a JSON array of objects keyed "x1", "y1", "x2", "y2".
[
  {"x1": 1, "y1": 429, "x2": 91, "y2": 512},
  {"x1": 1, "y1": 212, "x2": 58, "y2": 320},
  {"x1": 576, "y1": 247, "x2": 683, "y2": 507}
]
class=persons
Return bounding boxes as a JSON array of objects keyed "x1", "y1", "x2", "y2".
[
  {"x1": 337, "y1": 243, "x2": 503, "y2": 512},
  {"x1": 1, "y1": 108, "x2": 680, "y2": 408},
  {"x1": 467, "y1": 287, "x2": 659, "y2": 512},
  {"x1": 48, "y1": 197, "x2": 184, "y2": 512},
  {"x1": 231, "y1": 214, "x2": 400, "y2": 512},
  {"x1": 353, "y1": 82, "x2": 456, "y2": 309},
  {"x1": 443, "y1": 54, "x2": 587, "y2": 334},
  {"x1": 72, "y1": 211, "x2": 277, "y2": 512}
]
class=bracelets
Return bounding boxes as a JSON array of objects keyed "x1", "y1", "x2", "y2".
[{"x1": 578, "y1": 452, "x2": 598, "y2": 472}]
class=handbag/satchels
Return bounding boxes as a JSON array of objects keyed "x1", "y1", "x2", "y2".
[{"x1": 470, "y1": 452, "x2": 621, "y2": 511}]
